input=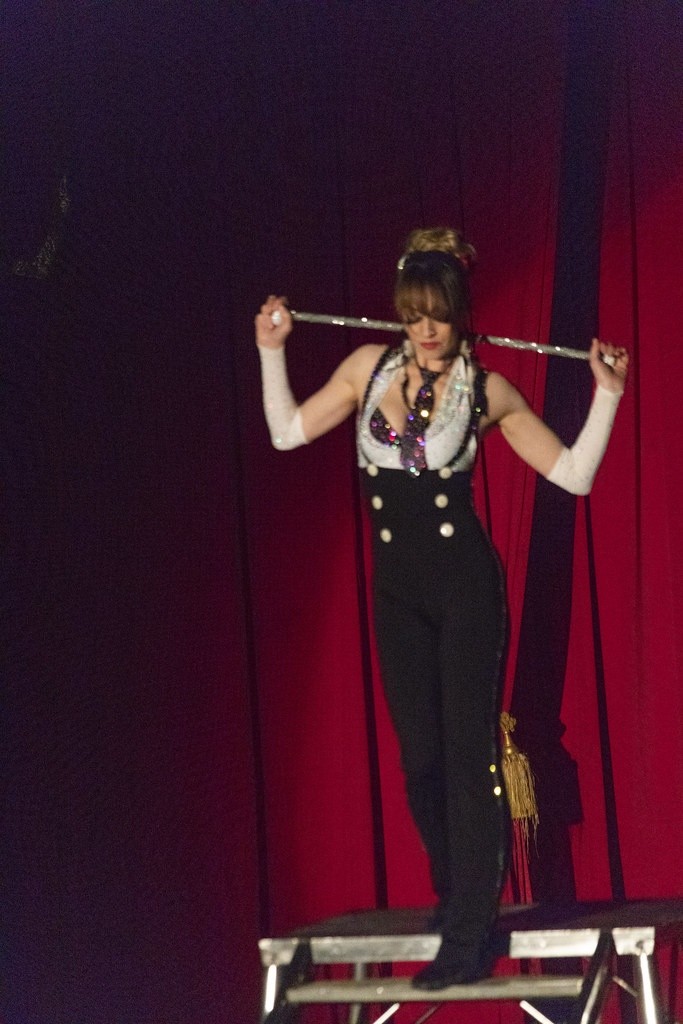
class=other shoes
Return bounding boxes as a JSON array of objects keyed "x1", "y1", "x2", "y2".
[{"x1": 412, "y1": 937, "x2": 491, "y2": 989}]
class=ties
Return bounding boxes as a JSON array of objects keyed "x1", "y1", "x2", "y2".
[{"x1": 399, "y1": 367, "x2": 441, "y2": 479}]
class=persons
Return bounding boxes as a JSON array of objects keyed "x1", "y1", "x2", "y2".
[{"x1": 255, "y1": 229, "x2": 630, "y2": 993}]
